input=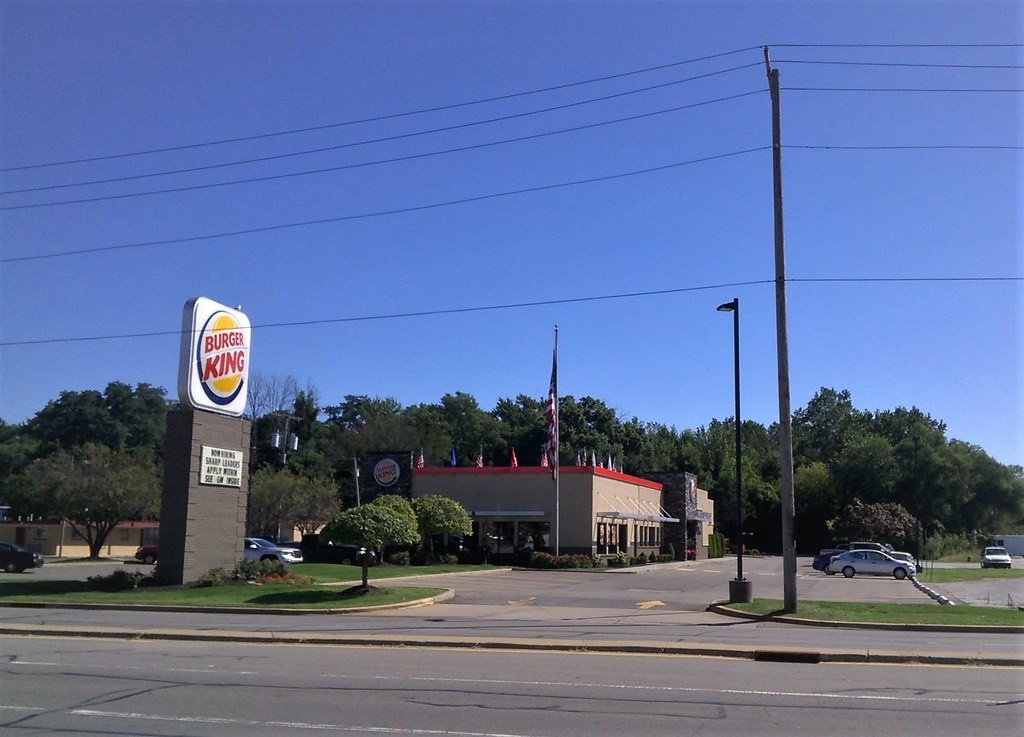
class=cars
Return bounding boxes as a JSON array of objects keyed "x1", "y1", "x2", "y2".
[
  {"x1": 301, "y1": 533, "x2": 378, "y2": 566},
  {"x1": 813, "y1": 550, "x2": 836, "y2": 575},
  {"x1": 828, "y1": 549, "x2": 916, "y2": 580},
  {"x1": 0, "y1": 541, "x2": 43, "y2": 573},
  {"x1": 245, "y1": 537, "x2": 304, "y2": 563},
  {"x1": 135, "y1": 542, "x2": 160, "y2": 565},
  {"x1": 980, "y1": 546, "x2": 1012, "y2": 569}
]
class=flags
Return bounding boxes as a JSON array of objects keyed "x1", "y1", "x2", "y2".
[
  {"x1": 511, "y1": 450, "x2": 518, "y2": 468},
  {"x1": 541, "y1": 443, "x2": 548, "y2": 467},
  {"x1": 546, "y1": 348, "x2": 557, "y2": 470},
  {"x1": 613, "y1": 461, "x2": 617, "y2": 472},
  {"x1": 451, "y1": 447, "x2": 457, "y2": 468},
  {"x1": 577, "y1": 453, "x2": 581, "y2": 466},
  {"x1": 582, "y1": 447, "x2": 587, "y2": 466},
  {"x1": 608, "y1": 455, "x2": 612, "y2": 471},
  {"x1": 417, "y1": 450, "x2": 424, "y2": 469},
  {"x1": 475, "y1": 450, "x2": 483, "y2": 468},
  {"x1": 620, "y1": 464, "x2": 623, "y2": 474},
  {"x1": 592, "y1": 452, "x2": 596, "y2": 467}
]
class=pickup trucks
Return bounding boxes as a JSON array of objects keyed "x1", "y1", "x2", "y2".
[{"x1": 820, "y1": 542, "x2": 915, "y2": 564}]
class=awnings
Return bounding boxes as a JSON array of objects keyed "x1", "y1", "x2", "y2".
[{"x1": 597, "y1": 512, "x2": 680, "y2": 523}]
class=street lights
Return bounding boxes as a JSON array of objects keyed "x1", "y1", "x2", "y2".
[{"x1": 716, "y1": 297, "x2": 743, "y2": 579}]
